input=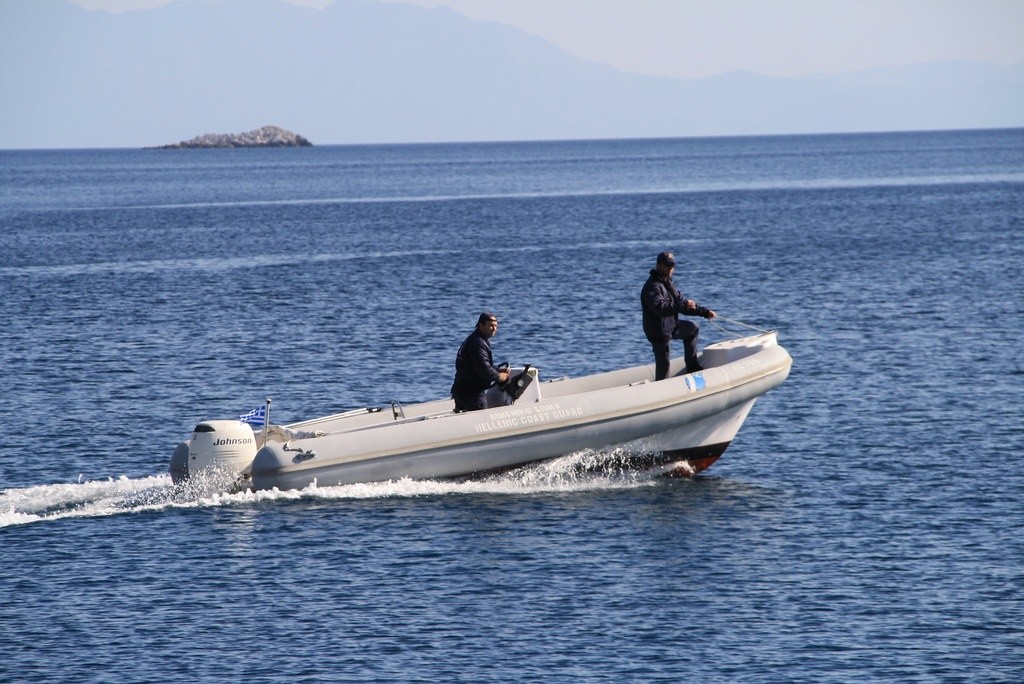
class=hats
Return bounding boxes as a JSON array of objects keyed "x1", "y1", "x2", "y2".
[
  {"x1": 474, "y1": 312, "x2": 497, "y2": 328},
  {"x1": 657, "y1": 251, "x2": 676, "y2": 267}
]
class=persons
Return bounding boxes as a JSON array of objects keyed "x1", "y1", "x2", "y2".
[
  {"x1": 639, "y1": 251, "x2": 717, "y2": 382},
  {"x1": 448, "y1": 312, "x2": 510, "y2": 413}
]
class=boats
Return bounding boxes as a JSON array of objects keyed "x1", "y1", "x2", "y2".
[{"x1": 167, "y1": 314, "x2": 794, "y2": 527}]
